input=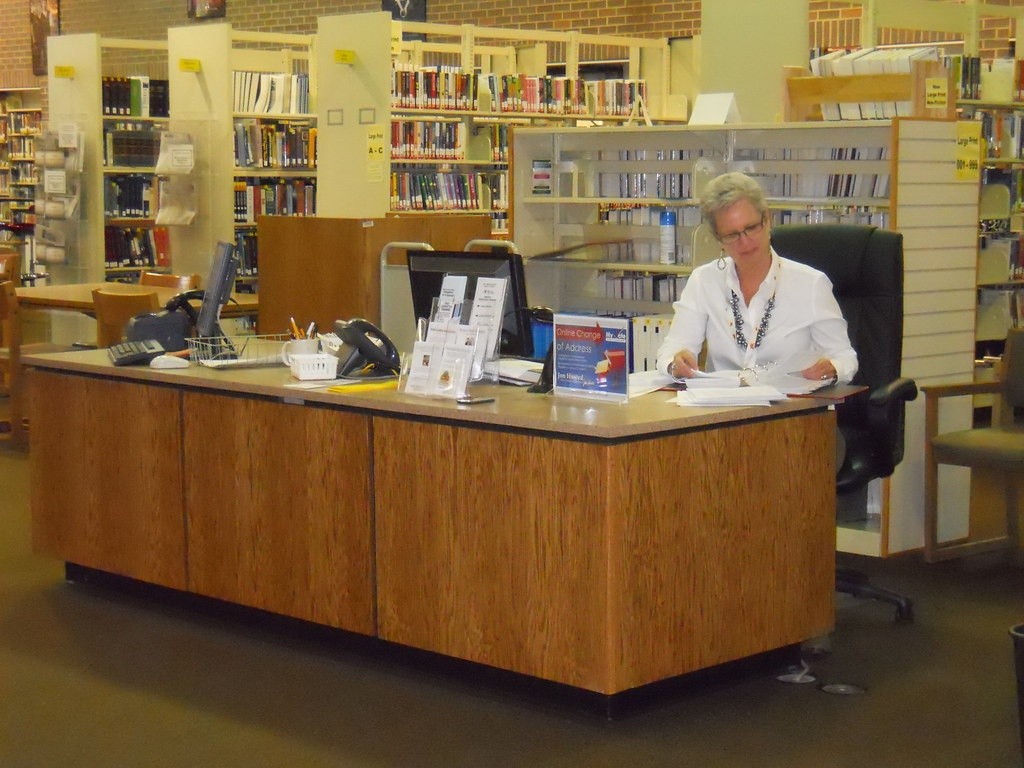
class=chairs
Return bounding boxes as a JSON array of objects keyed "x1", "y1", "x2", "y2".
[
  {"x1": 0, "y1": 254, "x2": 201, "y2": 450},
  {"x1": 766, "y1": 224, "x2": 918, "y2": 623},
  {"x1": 919, "y1": 328, "x2": 1024, "y2": 563}
]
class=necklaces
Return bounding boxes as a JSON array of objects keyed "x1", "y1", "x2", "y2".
[{"x1": 728, "y1": 275, "x2": 776, "y2": 351}]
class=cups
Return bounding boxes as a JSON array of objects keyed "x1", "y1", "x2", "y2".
[{"x1": 282, "y1": 339, "x2": 318, "y2": 365}]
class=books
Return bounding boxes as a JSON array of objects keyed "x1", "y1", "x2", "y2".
[
  {"x1": 102, "y1": 120, "x2": 171, "y2": 285},
  {"x1": 103, "y1": 75, "x2": 170, "y2": 118},
  {"x1": 0, "y1": 90, "x2": 44, "y2": 243},
  {"x1": 230, "y1": 71, "x2": 308, "y2": 115},
  {"x1": 390, "y1": 120, "x2": 508, "y2": 212},
  {"x1": 938, "y1": 54, "x2": 1024, "y2": 408},
  {"x1": 509, "y1": 121, "x2": 562, "y2": 127},
  {"x1": 812, "y1": 46, "x2": 938, "y2": 121},
  {"x1": 392, "y1": 60, "x2": 647, "y2": 117},
  {"x1": 535, "y1": 142, "x2": 892, "y2": 385},
  {"x1": 232, "y1": 118, "x2": 317, "y2": 331}
]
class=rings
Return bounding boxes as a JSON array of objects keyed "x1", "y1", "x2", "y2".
[{"x1": 822, "y1": 374, "x2": 827, "y2": 380}]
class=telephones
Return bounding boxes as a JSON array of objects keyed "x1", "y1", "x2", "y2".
[{"x1": 336, "y1": 318, "x2": 400, "y2": 373}]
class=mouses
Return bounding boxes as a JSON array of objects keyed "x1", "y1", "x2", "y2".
[{"x1": 150, "y1": 356, "x2": 190, "y2": 369}]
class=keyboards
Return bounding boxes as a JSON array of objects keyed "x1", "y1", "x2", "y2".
[{"x1": 108, "y1": 339, "x2": 166, "y2": 366}]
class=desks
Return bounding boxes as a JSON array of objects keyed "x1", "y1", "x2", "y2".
[
  {"x1": 18, "y1": 334, "x2": 843, "y2": 722},
  {"x1": 15, "y1": 283, "x2": 260, "y2": 321}
]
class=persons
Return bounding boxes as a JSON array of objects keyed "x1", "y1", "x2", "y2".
[{"x1": 655, "y1": 172, "x2": 859, "y2": 657}]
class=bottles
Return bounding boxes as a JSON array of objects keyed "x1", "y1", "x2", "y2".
[{"x1": 660, "y1": 211, "x2": 675, "y2": 265}]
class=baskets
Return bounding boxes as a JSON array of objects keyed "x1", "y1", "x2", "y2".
[{"x1": 183, "y1": 334, "x2": 290, "y2": 369}]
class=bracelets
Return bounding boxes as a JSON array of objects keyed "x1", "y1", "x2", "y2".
[{"x1": 670, "y1": 363, "x2": 688, "y2": 384}]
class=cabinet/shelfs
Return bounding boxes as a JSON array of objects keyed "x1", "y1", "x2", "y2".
[
  {"x1": 509, "y1": 57, "x2": 1024, "y2": 558},
  {"x1": 0, "y1": 10, "x2": 671, "y2": 346}
]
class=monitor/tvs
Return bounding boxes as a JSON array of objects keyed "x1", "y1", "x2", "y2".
[
  {"x1": 195, "y1": 238, "x2": 238, "y2": 362},
  {"x1": 407, "y1": 249, "x2": 535, "y2": 357}
]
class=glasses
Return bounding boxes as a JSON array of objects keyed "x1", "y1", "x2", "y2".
[{"x1": 712, "y1": 213, "x2": 763, "y2": 244}]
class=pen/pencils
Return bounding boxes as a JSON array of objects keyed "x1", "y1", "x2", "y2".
[{"x1": 286, "y1": 314, "x2": 318, "y2": 340}]
